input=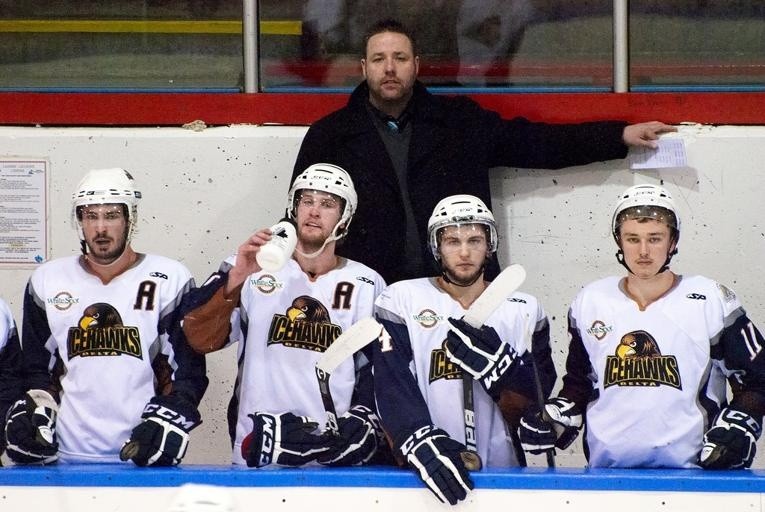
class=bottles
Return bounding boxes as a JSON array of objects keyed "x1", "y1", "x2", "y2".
[{"x1": 255, "y1": 216, "x2": 300, "y2": 270}]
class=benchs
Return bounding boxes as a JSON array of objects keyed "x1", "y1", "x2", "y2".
[
  {"x1": 265, "y1": 52, "x2": 763, "y2": 88},
  {"x1": 1, "y1": 14, "x2": 303, "y2": 39}
]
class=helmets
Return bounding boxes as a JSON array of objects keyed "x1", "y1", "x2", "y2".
[
  {"x1": 69, "y1": 165, "x2": 143, "y2": 244},
  {"x1": 610, "y1": 183, "x2": 681, "y2": 253},
  {"x1": 285, "y1": 162, "x2": 359, "y2": 241},
  {"x1": 426, "y1": 194, "x2": 499, "y2": 262}
]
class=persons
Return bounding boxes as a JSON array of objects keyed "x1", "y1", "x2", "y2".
[
  {"x1": 369, "y1": 193, "x2": 557, "y2": 506},
  {"x1": 0, "y1": 298, "x2": 25, "y2": 471},
  {"x1": 180, "y1": 162, "x2": 388, "y2": 469},
  {"x1": 22, "y1": 169, "x2": 209, "y2": 470},
  {"x1": 518, "y1": 185, "x2": 764, "y2": 470},
  {"x1": 284, "y1": 18, "x2": 679, "y2": 286}
]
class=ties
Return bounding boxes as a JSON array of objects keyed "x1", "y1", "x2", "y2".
[{"x1": 388, "y1": 119, "x2": 406, "y2": 134}]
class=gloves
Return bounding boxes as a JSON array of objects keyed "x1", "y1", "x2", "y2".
[
  {"x1": 2, "y1": 380, "x2": 64, "y2": 466},
  {"x1": 441, "y1": 315, "x2": 526, "y2": 401},
  {"x1": 696, "y1": 404, "x2": 763, "y2": 471},
  {"x1": 315, "y1": 401, "x2": 386, "y2": 467},
  {"x1": 117, "y1": 400, "x2": 204, "y2": 467},
  {"x1": 239, "y1": 410, "x2": 333, "y2": 471},
  {"x1": 394, "y1": 424, "x2": 483, "y2": 506},
  {"x1": 516, "y1": 395, "x2": 584, "y2": 455}
]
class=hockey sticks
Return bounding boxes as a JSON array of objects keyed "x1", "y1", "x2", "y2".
[
  {"x1": 314, "y1": 316, "x2": 381, "y2": 435},
  {"x1": 463, "y1": 264, "x2": 526, "y2": 452}
]
